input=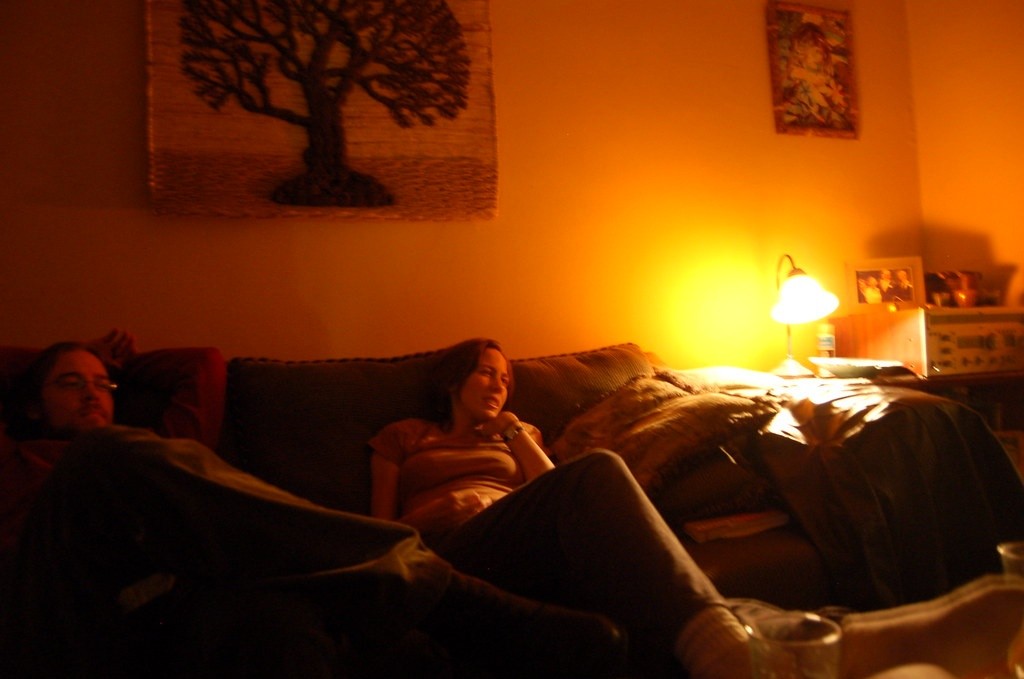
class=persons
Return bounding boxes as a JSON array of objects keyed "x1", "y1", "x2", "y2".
[
  {"x1": 367, "y1": 340, "x2": 851, "y2": 679},
  {"x1": 0, "y1": 329, "x2": 622, "y2": 679},
  {"x1": 859, "y1": 270, "x2": 911, "y2": 304}
]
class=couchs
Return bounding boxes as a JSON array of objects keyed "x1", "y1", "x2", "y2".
[{"x1": 0, "y1": 344, "x2": 1024, "y2": 679}]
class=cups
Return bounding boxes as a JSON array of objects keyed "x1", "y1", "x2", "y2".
[
  {"x1": 745, "y1": 614, "x2": 842, "y2": 679},
  {"x1": 997, "y1": 542, "x2": 1024, "y2": 576}
]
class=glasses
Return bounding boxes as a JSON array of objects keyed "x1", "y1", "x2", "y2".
[{"x1": 40, "y1": 371, "x2": 120, "y2": 392}]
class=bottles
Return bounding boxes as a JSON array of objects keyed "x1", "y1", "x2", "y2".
[{"x1": 817, "y1": 319, "x2": 836, "y2": 376}]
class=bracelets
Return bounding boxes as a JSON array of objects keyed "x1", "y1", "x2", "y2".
[{"x1": 503, "y1": 421, "x2": 523, "y2": 443}]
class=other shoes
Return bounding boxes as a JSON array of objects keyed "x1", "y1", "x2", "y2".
[{"x1": 419, "y1": 573, "x2": 630, "y2": 679}]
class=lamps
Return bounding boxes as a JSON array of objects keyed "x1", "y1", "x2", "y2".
[{"x1": 770, "y1": 254, "x2": 843, "y2": 379}]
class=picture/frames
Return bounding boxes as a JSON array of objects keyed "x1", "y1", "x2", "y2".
[
  {"x1": 764, "y1": 0, "x2": 863, "y2": 140},
  {"x1": 842, "y1": 255, "x2": 929, "y2": 304}
]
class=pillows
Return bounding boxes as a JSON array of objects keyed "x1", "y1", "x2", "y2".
[
  {"x1": 220, "y1": 349, "x2": 454, "y2": 517},
  {"x1": 549, "y1": 374, "x2": 777, "y2": 500},
  {"x1": 508, "y1": 343, "x2": 657, "y2": 453}
]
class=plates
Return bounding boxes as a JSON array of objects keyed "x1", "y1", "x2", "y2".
[{"x1": 809, "y1": 356, "x2": 904, "y2": 378}]
